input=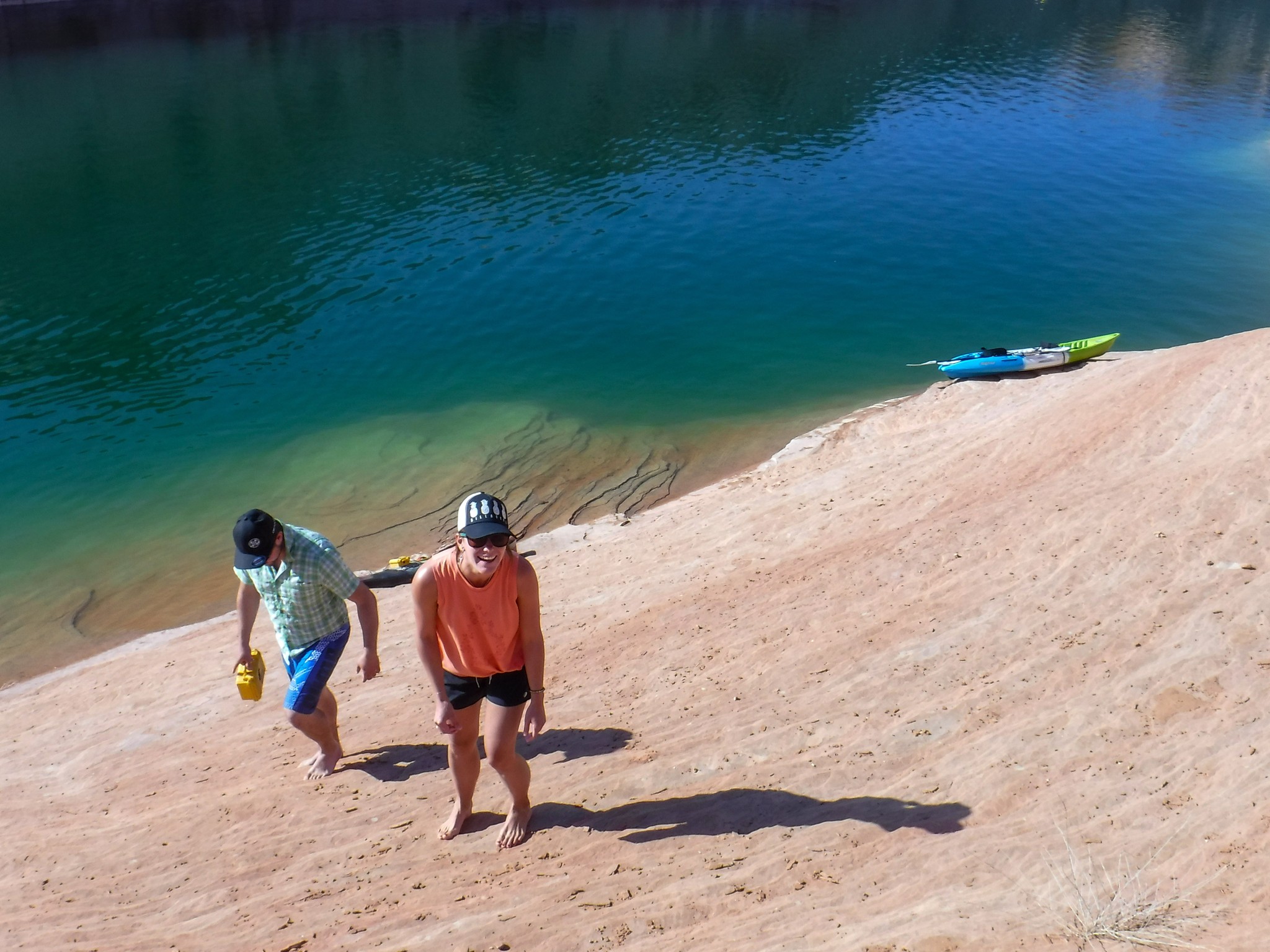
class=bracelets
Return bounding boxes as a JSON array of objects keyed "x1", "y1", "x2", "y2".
[{"x1": 529, "y1": 687, "x2": 546, "y2": 694}]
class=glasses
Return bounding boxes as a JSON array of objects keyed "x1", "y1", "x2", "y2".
[{"x1": 459, "y1": 532, "x2": 509, "y2": 548}]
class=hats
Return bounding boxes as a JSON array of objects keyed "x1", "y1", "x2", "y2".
[
  {"x1": 233, "y1": 509, "x2": 277, "y2": 570},
  {"x1": 457, "y1": 491, "x2": 517, "y2": 540}
]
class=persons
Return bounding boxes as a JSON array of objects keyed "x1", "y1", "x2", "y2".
[
  {"x1": 232, "y1": 508, "x2": 381, "y2": 780},
  {"x1": 412, "y1": 491, "x2": 547, "y2": 848}
]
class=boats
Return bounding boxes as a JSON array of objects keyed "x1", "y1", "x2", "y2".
[{"x1": 937, "y1": 331, "x2": 1120, "y2": 380}]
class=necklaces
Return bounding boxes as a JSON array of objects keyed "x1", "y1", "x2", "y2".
[{"x1": 458, "y1": 550, "x2": 463, "y2": 569}]
